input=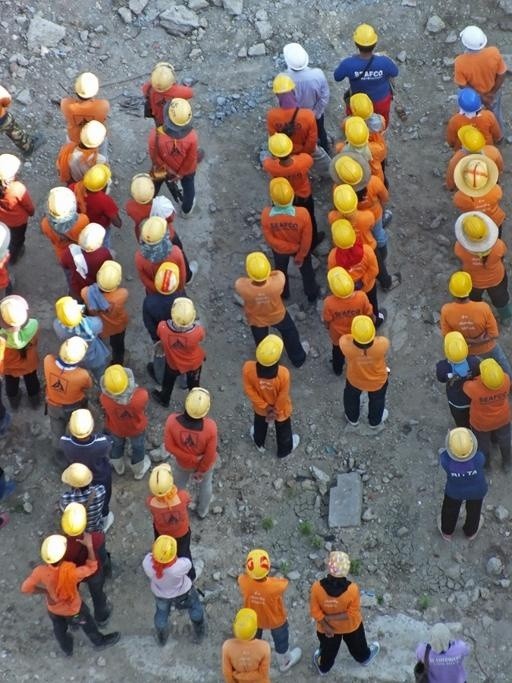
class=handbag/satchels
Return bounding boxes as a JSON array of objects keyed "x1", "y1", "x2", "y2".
[{"x1": 412, "y1": 659, "x2": 431, "y2": 683}]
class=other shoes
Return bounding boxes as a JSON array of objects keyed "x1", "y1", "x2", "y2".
[
  {"x1": 318, "y1": 288, "x2": 326, "y2": 297},
  {"x1": 10, "y1": 246, "x2": 25, "y2": 265},
  {"x1": 14, "y1": 387, "x2": 24, "y2": 410},
  {"x1": 300, "y1": 340, "x2": 308, "y2": 353},
  {"x1": 344, "y1": 412, "x2": 359, "y2": 424},
  {"x1": 151, "y1": 388, "x2": 169, "y2": 409},
  {"x1": 317, "y1": 231, "x2": 325, "y2": 244}
]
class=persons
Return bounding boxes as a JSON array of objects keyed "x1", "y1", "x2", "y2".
[
  {"x1": 20, "y1": 464, "x2": 202, "y2": 654},
  {"x1": 436, "y1": 25, "x2": 512, "y2": 538},
  {"x1": 237, "y1": 550, "x2": 302, "y2": 672},
  {"x1": 310, "y1": 551, "x2": 380, "y2": 674},
  {"x1": 0, "y1": 61, "x2": 216, "y2": 533},
  {"x1": 236, "y1": 42, "x2": 331, "y2": 458},
  {"x1": 323, "y1": 24, "x2": 397, "y2": 427},
  {"x1": 417, "y1": 621, "x2": 469, "y2": 682},
  {"x1": 221, "y1": 608, "x2": 272, "y2": 682}
]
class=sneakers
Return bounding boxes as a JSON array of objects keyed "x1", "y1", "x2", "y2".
[
  {"x1": 378, "y1": 306, "x2": 388, "y2": 321},
  {"x1": 191, "y1": 558, "x2": 205, "y2": 585},
  {"x1": 100, "y1": 509, "x2": 114, "y2": 535},
  {"x1": 249, "y1": 424, "x2": 266, "y2": 454},
  {"x1": 361, "y1": 640, "x2": 380, "y2": 667},
  {"x1": 368, "y1": 409, "x2": 388, "y2": 429},
  {"x1": 383, "y1": 208, "x2": 392, "y2": 227},
  {"x1": 466, "y1": 513, "x2": 485, "y2": 541},
  {"x1": 109, "y1": 455, "x2": 125, "y2": 477},
  {"x1": 96, "y1": 595, "x2": 113, "y2": 627},
  {"x1": 132, "y1": 454, "x2": 151, "y2": 482},
  {"x1": 382, "y1": 271, "x2": 403, "y2": 293},
  {"x1": 93, "y1": 631, "x2": 121, "y2": 652},
  {"x1": 291, "y1": 432, "x2": 300, "y2": 453},
  {"x1": 436, "y1": 511, "x2": 453, "y2": 542},
  {"x1": 312, "y1": 647, "x2": 329, "y2": 677}
]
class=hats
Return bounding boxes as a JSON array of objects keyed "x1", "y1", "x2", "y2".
[
  {"x1": 322, "y1": 549, "x2": 356, "y2": 583},
  {"x1": 452, "y1": 152, "x2": 500, "y2": 199}
]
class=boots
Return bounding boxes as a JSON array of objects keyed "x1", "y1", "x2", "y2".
[
  {"x1": 189, "y1": 611, "x2": 209, "y2": 646},
  {"x1": 272, "y1": 644, "x2": 303, "y2": 673},
  {"x1": 497, "y1": 301, "x2": 512, "y2": 327},
  {"x1": 155, "y1": 621, "x2": 173, "y2": 646}
]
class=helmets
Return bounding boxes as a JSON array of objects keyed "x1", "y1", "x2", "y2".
[
  {"x1": 38, "y1": 532, "x2": 70, "y2": 566},
  {"x1": 478, "y1": 355, "x2": 506, "y2": 393},
  {"x1": 457, "y1": 87, "x2": 482, "y2": 113},
  {"x1": 59, "y1": 500, "x2": 90, "y2": 540},
  {"x1": 447, "y1": 426, "x2": 476, "y2": 461},
  {"x1": 0, "y1": 154, "x2": 21, "y2": 179},
  {"x1": 353, "y1": 25, "x2": 376, "y2": 47},
  {"x1": 0, "y1": 294, "x2": 29, "y2": 327},
  {"x1": 151, "y1": 534, "x2": 178, "y2": 565},
  {"x1": 186, "y1": 388, "x2": 211, "y2": 420},
  {"x1": 1, "y1": 223, "x2": 11, "y2": 251},
  {"x1": 243, "y1": 545, "x2": 275, "y2": 584},
  {"x1": 269, "y1": 43, "x2": 309, "y2": 207},
  {"x1": 454, "y1": 208, "x2": 499, "y2": 255},
  {"x1": 47, "y1": 64, "x2": 195, "y2": 394},
  {"x1": 148, "y1": 462, "x2": 175, "y2": 500},
  {"x1": 442, "y1": 329, "x2": 471, "y2": 366},
  {"x1": 448, "y1": 269, "x2": 473, "y2": 299},
  {"x1": 231, "y1": 605, "x2": 260, "y2": 647},
  {"x1": 66, "y1": 408, "x2": 96, "y2": 441},
  {"x1": 255, "y1": 334, "x2": 285, "y2": 367},
  {"x1": 244, "y1": 251, "x2": 271, "y2": 282},
  {"x1": 459, "y1": 25, "x2": 488, "y2": 53},
  {"x1": 327, "y1": 92, "x2": 375, "y2": 343},
  {"x1": 456, "y1": 124, "x2": 486, "y2": 154},
  {"x1": 61, "y1": 462, "x2": 95, "y2": 490}
]
